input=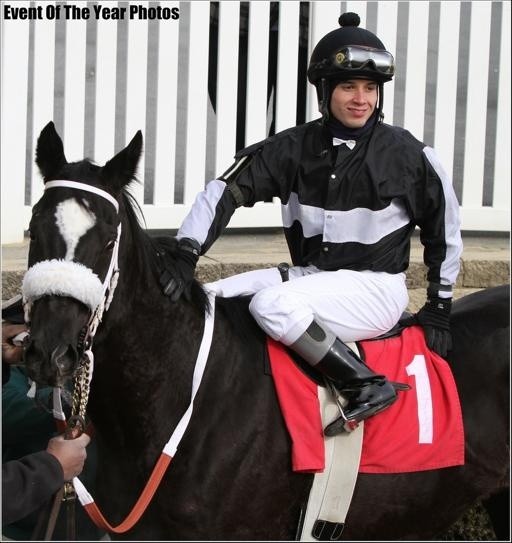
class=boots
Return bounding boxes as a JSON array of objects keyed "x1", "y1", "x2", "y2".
[{"x1": 287, "y1": 319, "x2": 399, "y2": 436}]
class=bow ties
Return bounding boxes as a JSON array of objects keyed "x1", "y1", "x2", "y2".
[{"x1": 332, "y1": 137, "x2": 357, "y2": 151}]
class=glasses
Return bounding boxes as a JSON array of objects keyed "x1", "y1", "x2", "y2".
[{"x1": 309, "y1": 42, "x2": 395, "y2": 77}]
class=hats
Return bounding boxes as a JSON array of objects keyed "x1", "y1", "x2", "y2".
[{"x1": 305, "y1": 11, "x2": 395, "y2": 82}]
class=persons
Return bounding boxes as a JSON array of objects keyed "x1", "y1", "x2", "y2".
[
  {"x1": 146, "y1": 11, "x2": 465, "y2": 442},
  {"x1": 1, "y1": 291, "x2": 95, "y2": 535}
]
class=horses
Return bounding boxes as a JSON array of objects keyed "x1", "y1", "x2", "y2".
[{"x1": 21, "y1": 120, "x2": 511, "y2": 541}]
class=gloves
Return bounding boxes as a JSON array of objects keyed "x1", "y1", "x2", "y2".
[
  {"x1": 396, "y1": 300, "x2": 454, "y2": 357},
  {"x1": 156, "y1": 235, "x2": 203, "y2": 303}
]
